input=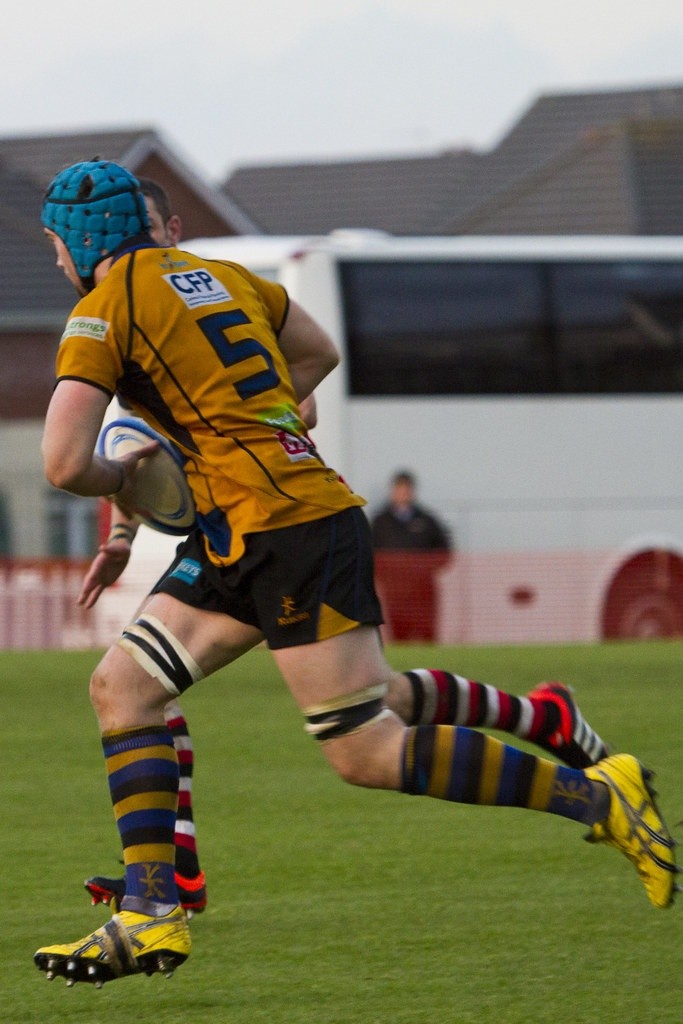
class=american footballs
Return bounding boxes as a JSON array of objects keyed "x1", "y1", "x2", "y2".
[{"x1": 97, "y1": 416, "x2": 199, "y2": 536}]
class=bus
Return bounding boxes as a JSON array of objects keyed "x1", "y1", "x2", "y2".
[{"x1": 103, "y1": 230, "x2": 682, "y2": 640}]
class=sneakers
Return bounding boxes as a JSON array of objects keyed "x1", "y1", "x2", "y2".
[
  {"x1": 529, "y1": 680, "x2": 608, "y2": 771},
  {"x1": 33, "y1": 901, "x2": 192, "y2": 988},
  {"x1": 581, "y1": 753, "x2": 681, "y2": 909},
  {"x1": 83, "y1": 860, "x2": 207, "y2": 914}
]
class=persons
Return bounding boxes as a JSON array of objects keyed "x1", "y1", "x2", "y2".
[
  {"x1": 72, "y1": 177, "x2": 612, "y2": 918},
  {"x1": 31, "y1": 156, "x2": 682, "y2": 989},
  {"x1": 371, "y1": 472, "x2": 452, "y2": 551}
]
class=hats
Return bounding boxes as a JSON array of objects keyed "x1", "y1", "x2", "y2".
[{"x1": 40, "y1": 159, "x2": 151, "y2": 287}]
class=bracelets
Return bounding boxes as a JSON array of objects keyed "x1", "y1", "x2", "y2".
[
  {"x1": 108, "y1": 459, "x2": 125, "y2": 494},
  {"x1": 108, "y1": 524, "x2": 135, "y2": 544}
]
